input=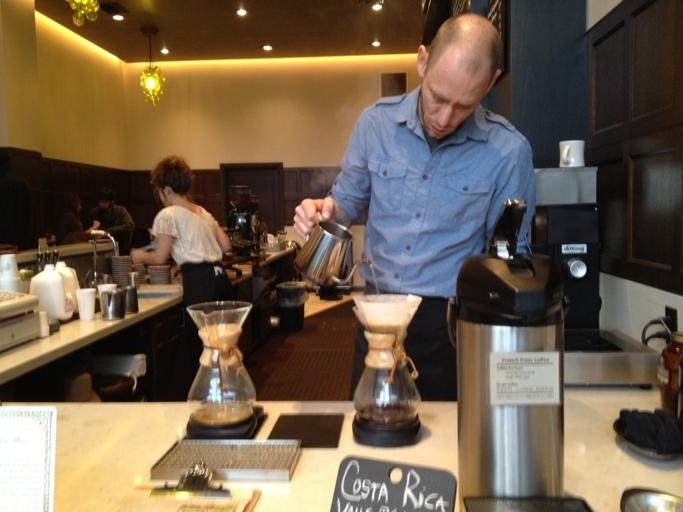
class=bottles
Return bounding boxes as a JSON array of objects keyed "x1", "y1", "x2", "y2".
[{"x1": 657, "y1": 332, "x2": 683, "y2": 416}]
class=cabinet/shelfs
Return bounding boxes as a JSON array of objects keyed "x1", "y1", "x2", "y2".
[{"x1": 421, "y1": 0, "x2": 586, "y2": 170}]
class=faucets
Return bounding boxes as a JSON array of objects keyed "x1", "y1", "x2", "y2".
[{"x1": 90, "y1": 230, "x2": 118, "y2": 280}]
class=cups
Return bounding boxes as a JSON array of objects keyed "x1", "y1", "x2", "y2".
[
  {"x1": 37, "y1": 311, "x2": 50, "y2": 338},
  {"x1": 1, "y1": 254, "x2": 21, "y2": 293},
  {"x1": 76, "y1": 283, "x2": 138, "y2": 320},
  {"x1": 558, "y1": 140, "x2": 586, "y2": 168}
]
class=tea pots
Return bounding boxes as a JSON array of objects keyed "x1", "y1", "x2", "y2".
[{"x1": 293, "y1": 209, "x2": 374, "y2": 290}]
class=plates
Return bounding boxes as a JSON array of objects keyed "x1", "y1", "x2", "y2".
[{"x1": 112, "y1": 256, "x2": 177, "y2": 285}]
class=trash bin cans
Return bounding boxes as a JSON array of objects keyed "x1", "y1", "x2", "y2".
[{"x1": 274, "y1": 281, "x2": 306, "y2": 332}]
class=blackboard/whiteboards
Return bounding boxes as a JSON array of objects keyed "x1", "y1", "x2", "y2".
[{"x1": 330, "y1": 457, "x2": 457, "y2": 512}]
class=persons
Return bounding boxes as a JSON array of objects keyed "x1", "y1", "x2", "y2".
[
  {"x1": 53, "y1": 192, "x2": 101, "y2": 247},
  {"x1": 129, "y1": 156, "x2": 238, "y2": 299},
  {"x1": 291, "y1": 12, "x2": 538, "y2": 406},
  {"x1": 89, "y1": 186, "x2": 134, "y2": 255}
]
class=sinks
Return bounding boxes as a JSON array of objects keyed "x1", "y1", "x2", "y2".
[{"x1": 136, "y1": 291, "x2": 179, "y2": 299}]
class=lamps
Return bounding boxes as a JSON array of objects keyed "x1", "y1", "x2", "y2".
[
  {"x1": 66, "y1": 0, "x2": 100, "y2": 28},
  {"x1": 139, "y1": 24, "x2": 166, "y2": 109}
]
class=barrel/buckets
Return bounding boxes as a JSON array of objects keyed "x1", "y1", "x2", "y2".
[
  {"x1": 56, "y1": 261, "x2": 80, "y2": 313},
  {"x1": 29, "y1": 262, "x2": 73, "y2": 321}
]
